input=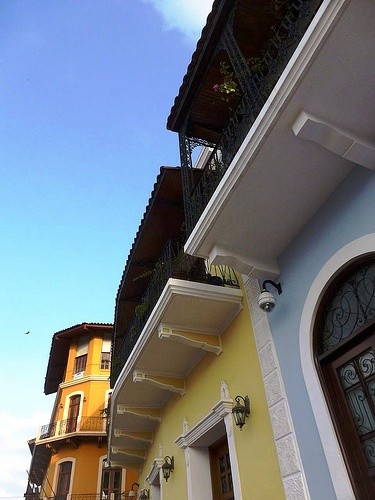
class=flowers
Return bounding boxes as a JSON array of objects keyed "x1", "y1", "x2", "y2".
[{"x1": 213, "y1": 61, "x2": 237, "y2": 100}]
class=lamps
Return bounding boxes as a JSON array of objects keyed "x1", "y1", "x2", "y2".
[
  {"x1": 232, "y1": 395, "x2": 250, "y2": 431},
  {"x1": 162, "y1": 455, "x2": 175, "y2": 482},
  {"x1": 257, "y1": 279, "x2": 282, "y2": 312},
  {"x1": 103, "y1": 459, "x2": 110, "y2": 468},
  {"x1": 129, "y1": 483, "x2": 139, "y2": 499}
]
